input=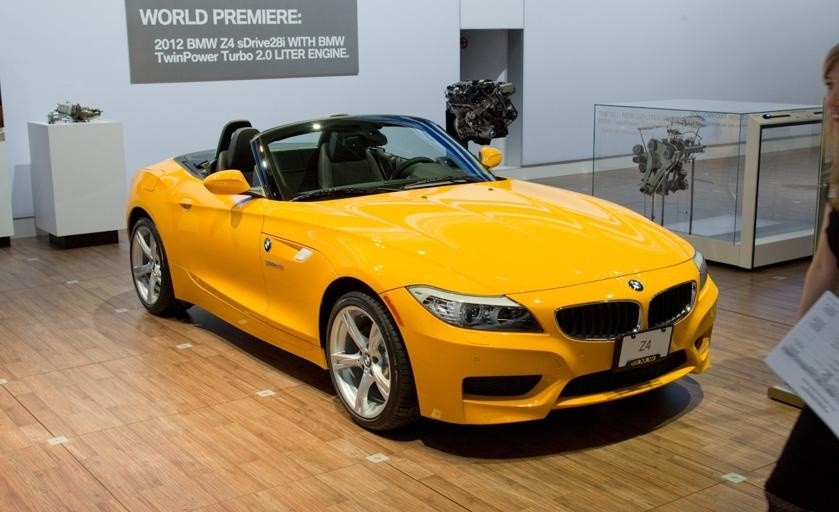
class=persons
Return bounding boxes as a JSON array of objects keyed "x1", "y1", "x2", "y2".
[{"x1": 765, "y1": 44, "x2": 839, "y2": 511}]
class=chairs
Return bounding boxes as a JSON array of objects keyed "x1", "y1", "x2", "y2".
[
  {"x1": 318, "y1": 127, "x2": 386, "y2": 190},
  {"x1": 214, "y1": 127, "x2": 261, "y2": 188}
]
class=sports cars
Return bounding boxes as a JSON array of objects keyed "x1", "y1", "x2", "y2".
[{"x1": 124, "y1": 113, "x2": 720, "y2": 432}]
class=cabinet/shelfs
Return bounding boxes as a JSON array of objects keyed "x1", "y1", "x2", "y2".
[{"x1": 591, "y1": 97, "x2": 823, "y2": 272}]
